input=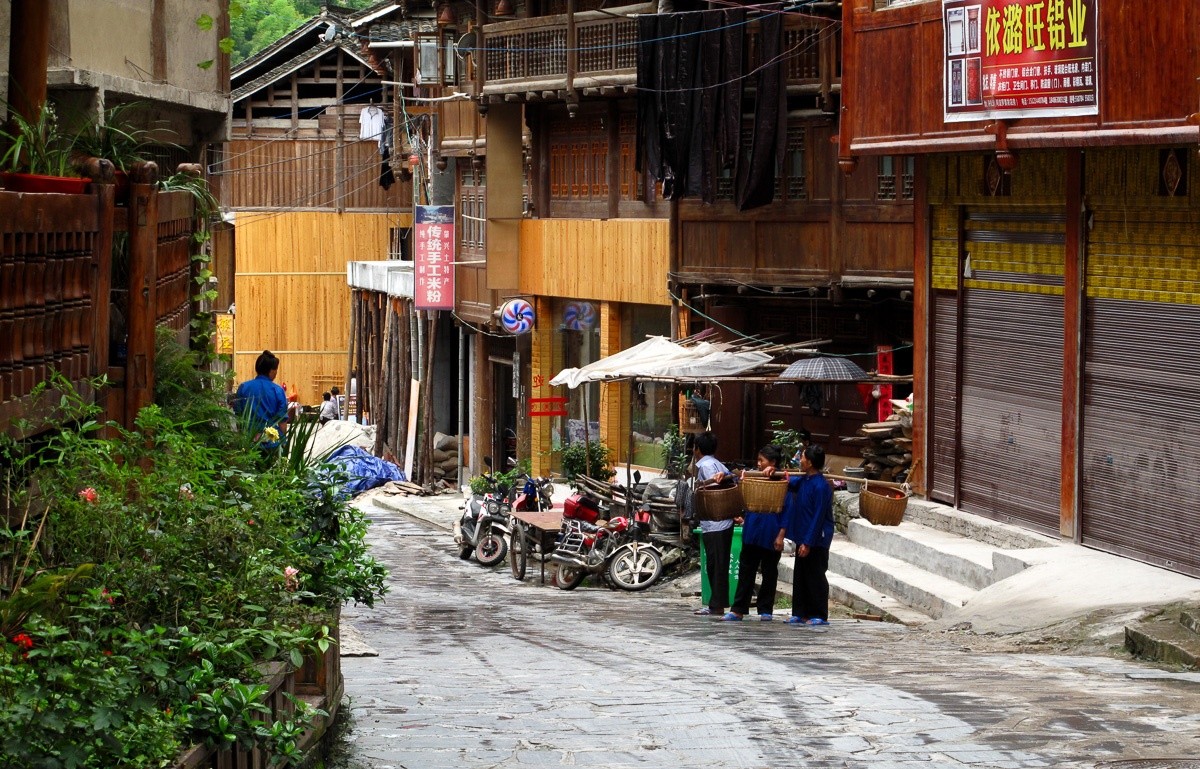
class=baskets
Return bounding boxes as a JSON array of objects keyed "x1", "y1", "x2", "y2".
[
  {"x1": 860, "y1": 479, "x2": 909, "y2": 526},
  {"x1": 738, "y1": 477, "x2": 789, "y2": 514},
  {"x1": 694, "y1": 483, "x2": 739, "y2": 522}
]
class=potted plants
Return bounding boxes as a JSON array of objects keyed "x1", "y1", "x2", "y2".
[{"x1": 659, "y1": 423, "x2": 689, "y2": 479}]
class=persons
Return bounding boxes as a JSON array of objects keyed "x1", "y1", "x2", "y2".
[
  {"x1": 235, "y1": 350, "x2": 289, "y2": 459},
  {"x1": 693, "y1": 433, "x2": 834, "y2": 625},
  {"x1": 320, "y1": 386, "x2": 340, "y2": 427}
]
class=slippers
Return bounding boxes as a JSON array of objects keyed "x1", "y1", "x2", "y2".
[
  {"x1": 720, "y1": 612, "x2": 742, "y2": 621},
  {"x1": 783, "y1": 615, "x2": 805, "y2": 623},
  {"x1": 693, "y1": 606, "x2": 724, "y2": 615},
  {"x1": 761, "y1": 613, "x2": 772, "y2": 621},
  {"x1": 807, "y1": 617, "x2": 830, "y2": 625}
]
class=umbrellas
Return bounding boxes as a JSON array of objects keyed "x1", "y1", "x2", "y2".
[{"x1": 778, "y1": 357, "x2": 867, "y2": 416}]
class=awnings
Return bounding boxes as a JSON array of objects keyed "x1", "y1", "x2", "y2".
[{"x1": 548, "y1": 334, "x2": 775, "y2": 478}]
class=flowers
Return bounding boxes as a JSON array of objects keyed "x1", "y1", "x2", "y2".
[
  {"x1": 0, "y1": 328, "x2": 390, "y2": 769},
  {"x1": 469, "y1": 457, "x2": 530, "y2": 496}
]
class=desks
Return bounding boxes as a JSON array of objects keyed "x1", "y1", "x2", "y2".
[{"x1": 507, "y1": 511, "x2": 564, "y2": 584}]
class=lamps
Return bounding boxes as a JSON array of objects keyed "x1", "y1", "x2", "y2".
[{"x1": 492, "y1": 294, "x2": 537, "y2": 336}]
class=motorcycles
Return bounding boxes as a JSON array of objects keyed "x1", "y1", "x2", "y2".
[
  {"x1": 451, "y1": 456, "x2": 513, "y2": 566},
  {"x1": 503, "y1": 455, "x2": 555, "y2": 563},
  {"x1": 550, "y1": 469, "x2": 669, "y2": 592}
]
